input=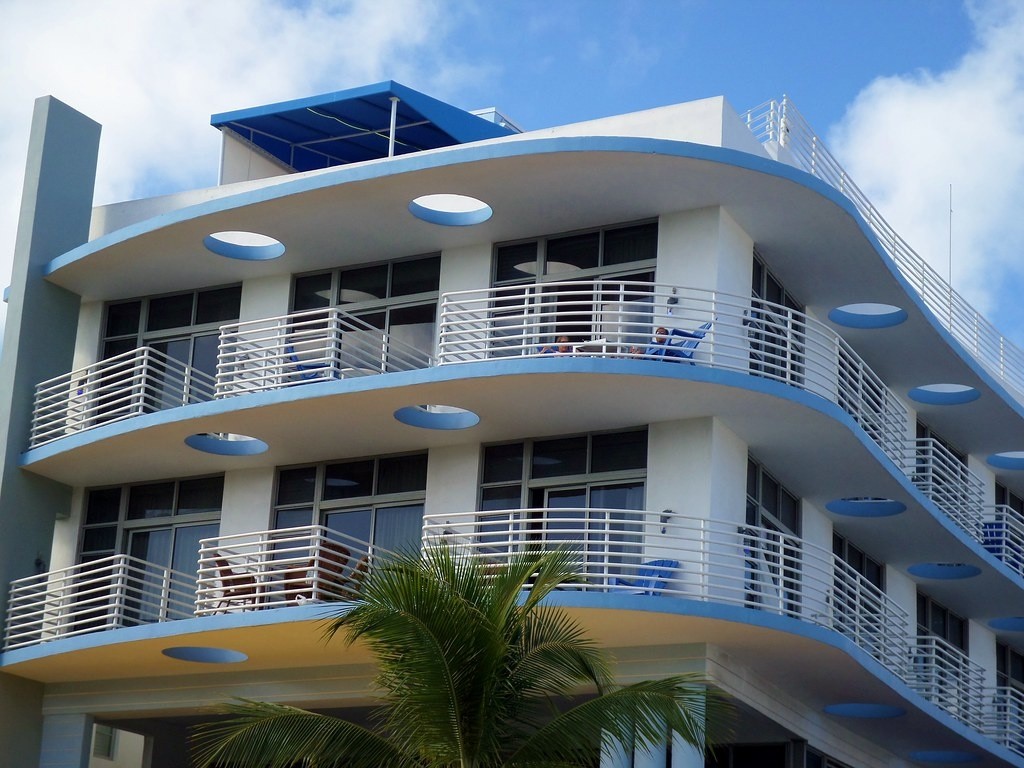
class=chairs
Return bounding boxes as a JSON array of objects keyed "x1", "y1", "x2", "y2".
[
  {"x1": 974, "y1": 521, "x2": 1021, "y2": 574},
  {"x1": 534, "y1": 343, "x2": 574, "y2": 358},
  {"x1": 646, "y1": 340, "x2": 686, "y2": 362},
  {"x1": 287, "y1": 339, "x2": 331, "y2": 387},
  {"x1": 643, "y1": 317, "x2": 718, "y2": 366},
  {"x1": 284, "y1": 543, "x2": 350, "y2": 606},
  {"x1": 335, "y1": 555, "x2": 369, "y2": 602},
  {"x1": 608, "y1": 559, "x2": 679, "y2": 597},
  {"x1": 212, "y1": 553, "x2": 265, "y2": 616}
]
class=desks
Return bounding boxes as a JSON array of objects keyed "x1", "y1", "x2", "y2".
[
  {"x1": 573, "y1": 341, "x2": 628, "y2": 359},
  {"x1": 339, "y1": 368, "x2": 381, "y2": 379}
]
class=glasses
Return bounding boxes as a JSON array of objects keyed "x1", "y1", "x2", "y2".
[{"x1": 656, "y1": 331, "x2": 665, "y2": 335}]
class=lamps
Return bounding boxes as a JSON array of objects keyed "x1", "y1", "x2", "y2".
[
  {"x1": 743, "y1": 310, "x2": 757, "y2": 338},
  {"x1": 238, "y1": 354, "x2": 250, "y2": 376},
  {"x1": 738, "y1": 527, "x2": 752, "y2": 554},
  {"x1": 77, "y1": 379, "x2": 87, "y2": 395},
  {"x1": 667, "y1": 288, "x2": 678, "y2": 315},
  {"x1": 439, "y1": 521, "x2": 451, "y2": 552},
  {"x1": 660, "y1": 510, "x2": 677, "y2": 534}
]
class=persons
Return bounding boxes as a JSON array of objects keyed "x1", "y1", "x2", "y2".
[
  {"x1": 629, "y1": 327, "x2": 669, "y2": 360},
  {"x1": 538, "y1": 336, "x2": 575, "y2": 358}
]
